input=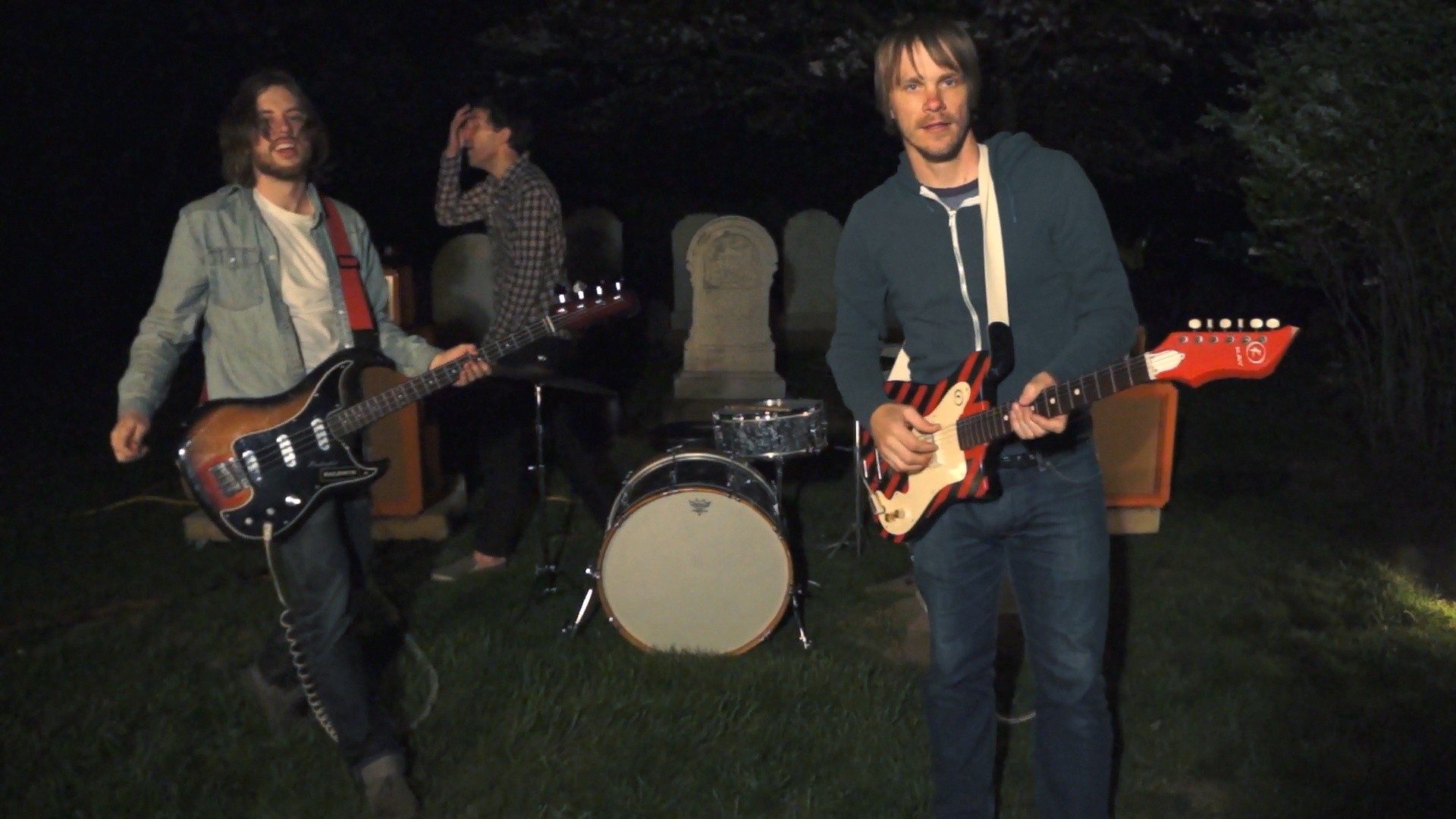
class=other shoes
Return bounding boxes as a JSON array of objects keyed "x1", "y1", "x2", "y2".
[
  {"x1": 238, "y1": 619, "x2": 306, "y2": 730},
  {"x1": 362, "y1": 756, "x2": 418, "y2": 818},
  {"x1": 429, "y1": 550, "x2": 508, "y2": 582}
]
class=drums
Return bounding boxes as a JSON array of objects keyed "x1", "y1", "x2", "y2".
[
  {"x1": 595, "y1": 450, "x2": 795, "y2": 658},
  {"x1": 712, "y1": 398, "x2": 828, "y2": 461}
]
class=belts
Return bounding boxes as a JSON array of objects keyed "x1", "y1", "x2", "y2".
[{"x1": 990, "y1": 414, "x2": 1094, "y2": 468}]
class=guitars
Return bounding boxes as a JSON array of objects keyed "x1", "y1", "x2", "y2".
[
  {"x1": 859, "y1": 316, "x2": 1300, "y2": 545},
  {"x1": 174, "y1": 280, "x2": 629, "y2": 544}
]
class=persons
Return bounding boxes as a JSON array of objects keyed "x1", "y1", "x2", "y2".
[
  {"x1": 110, "y1": 67, "x2": 496, "y2": 819},
  {"x1": 435, "y1": 97, "x2": 563, "y2": 578},
  {"x1": 827, "y1": 19, "x2": 1141, "y2": 819}
]
